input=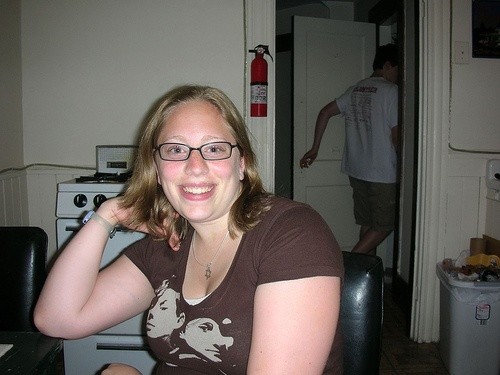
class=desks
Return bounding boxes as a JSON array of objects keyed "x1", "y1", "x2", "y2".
[{"x1": 0, "y1": 332, "x2": 63, "y2": 375}]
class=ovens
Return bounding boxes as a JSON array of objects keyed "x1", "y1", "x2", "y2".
[{"x1": 55, "y1": 219, "x2": 157, "y2": 375}]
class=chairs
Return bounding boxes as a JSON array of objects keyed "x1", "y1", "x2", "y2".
[
  {"x1": 0, "y1": 226, "x2": 48, "y2": 330},
  {"x1": 338, "y1": 251, "x2": 384, "y2": 375}
]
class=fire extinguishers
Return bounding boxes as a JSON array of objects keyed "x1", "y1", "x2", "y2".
[{"x1": 248, "y1": 44, "x2": 273, "y2": 118}]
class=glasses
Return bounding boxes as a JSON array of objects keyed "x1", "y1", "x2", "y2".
[{"x1": 153, "y1": 142, "x2": 239, "y2": 161}]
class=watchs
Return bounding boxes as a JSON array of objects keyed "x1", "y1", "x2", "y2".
[{"x1": 83, "y1": 209, "x2": 117, "y2": 238}]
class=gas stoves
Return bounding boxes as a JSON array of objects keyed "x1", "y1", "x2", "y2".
[{"x1": 56, "y1": 145, "x2": 140, "y2": 218}]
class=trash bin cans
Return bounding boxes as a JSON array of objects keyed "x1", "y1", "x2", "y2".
[{"x1": 436, "y1": 260, "x2": 499, "y2": 374}]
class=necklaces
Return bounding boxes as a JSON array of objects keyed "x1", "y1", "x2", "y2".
[{"x1": 192, "y1": 229, "x2": 230, "y2": 280}]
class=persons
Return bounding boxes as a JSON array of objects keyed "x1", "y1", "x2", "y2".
[
  {"x1": 298, "y1": 42, "x2": 399, "y2": 283},
  {"x1": 32, "y1": 84, "x2": 345, "y2": 375}
]
